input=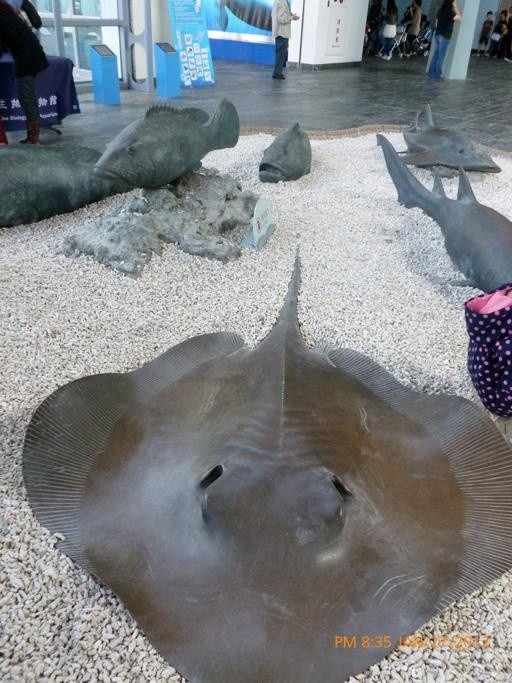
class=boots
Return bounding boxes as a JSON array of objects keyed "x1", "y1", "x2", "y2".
[
  {"x1": 0, "y1": 116, "x2": 9, "y2": 145},
  {"x1": 19, "y1": 120, "x2": 40, "y2": 146}
]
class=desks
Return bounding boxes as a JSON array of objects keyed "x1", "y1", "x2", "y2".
[{"x1": 0, "y1": 54, "x2": 74, "y2": 136}]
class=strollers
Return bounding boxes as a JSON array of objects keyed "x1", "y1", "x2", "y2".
[
  {"x1": 410, "y1": 25, "x2": 433, "y2": 57},
  {"x1": 375, "y1": 23, "x2": 408, "y2": 59}
]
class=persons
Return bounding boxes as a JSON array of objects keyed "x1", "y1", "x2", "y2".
[
  {"x1": 426, "y1": 0, "x2": 462, "y2": 80},
  {"x1": 464, "y1": 283, "x2": 512, "y2": 418},
  {"x1": 364, "y1": 0, "x2": 432, "y2": 61},
  {"x1": 271, "y1": 0, "x2": 300, "y2": 79},
  {"x1": 476, "y1": 7, "x2": 512, "y2": 61},
  {"x1": 0, "y1": 0, "x2": 42, "y2": 145}
]
